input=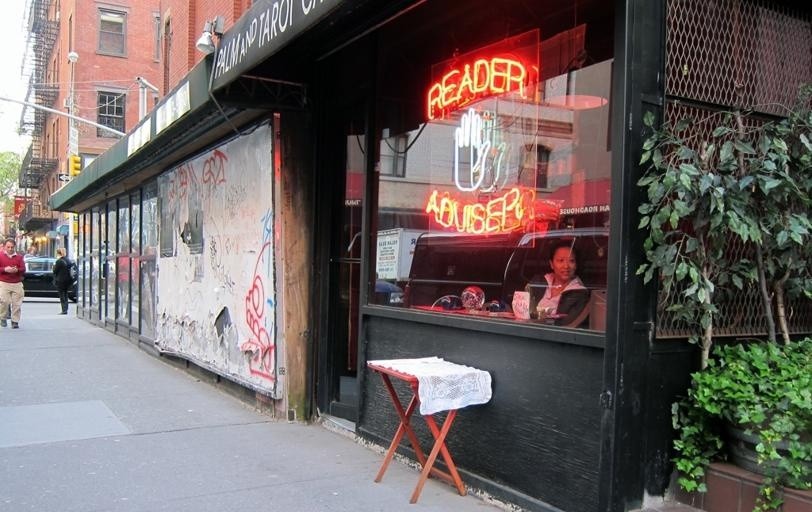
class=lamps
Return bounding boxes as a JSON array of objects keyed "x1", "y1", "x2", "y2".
[
  {"x1": 68, "y1": 49, "x2": 81, "y2": 68},
  {"x1": 195, "y1": 11, "x2": 227, "y2": 58}
]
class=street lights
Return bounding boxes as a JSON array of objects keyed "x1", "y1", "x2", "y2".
[{"x1": 67, "y1": 49, "x2": 79, "y2": 183}]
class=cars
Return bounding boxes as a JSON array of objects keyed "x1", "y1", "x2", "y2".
[
  {"x1": 77, "y1": 263, "x2": 115, "y2": 304},
  {"x1": 20, "y1": 252, "x2": 78, "y2": 302},
  {"x1": 375, "y1": 271, "x2": 404, "y2": 307}
]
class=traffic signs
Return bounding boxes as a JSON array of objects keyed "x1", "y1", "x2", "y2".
[
  {"x1": 57, "y1": 173, "x2": 68, "y2": 182},
  {"x1": 68, "y1": 125, "x2": 78, "y2": 155}
]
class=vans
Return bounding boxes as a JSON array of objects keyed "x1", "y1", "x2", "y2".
[
  {"x1": 402, "y1": 231, "x2": 531, "y2": 311},
  {"x1": 499, "y1": 227, "x2": 608, "y2": 319}
]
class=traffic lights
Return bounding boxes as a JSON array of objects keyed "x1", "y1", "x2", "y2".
[{"x1": 69, "y1": 154, "x2": 81, "y2": 177}]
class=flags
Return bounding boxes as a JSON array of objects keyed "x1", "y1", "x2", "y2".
[{"x1": 14, "y1": 196, "x2": 32, "y2": 215}]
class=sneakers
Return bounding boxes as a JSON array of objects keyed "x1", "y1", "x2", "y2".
[
  {"x1": 0, "y1": 320, "x2": 6, "y2": 327},
  {"x1": 12, "y1": 322, "x2": 18, "y2": 328}
]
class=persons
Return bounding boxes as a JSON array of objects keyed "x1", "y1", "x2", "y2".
[
  {"x1": 52, "y1": 247, "x2": 77, "y2": 315},
  {"x1": 0, "y1": 239, "x2": 26, "y2": 329},
  {"x1": 523, "y1": 240, "x2": 592, "y2": 325},
  {"x1": 0, "y1": 240, "x2": 12, "y2": 319}
]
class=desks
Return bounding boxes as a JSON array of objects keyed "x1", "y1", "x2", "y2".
[{"x1": 364, "y1": 349, "x2": 500, "y2": 506}]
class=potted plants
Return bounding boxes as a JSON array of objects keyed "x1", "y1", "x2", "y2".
[{"x1": 666, "y1": 326, "x2": 812, "y2": 509}]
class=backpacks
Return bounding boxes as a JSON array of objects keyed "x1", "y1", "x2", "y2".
[{"x1": 61, "y1": 258, "x2": 78, "y2": 282}]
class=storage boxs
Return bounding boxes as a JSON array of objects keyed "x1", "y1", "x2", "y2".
[{"x1": 376, "y1": 228, "x2": 430, "y2": 281}]
